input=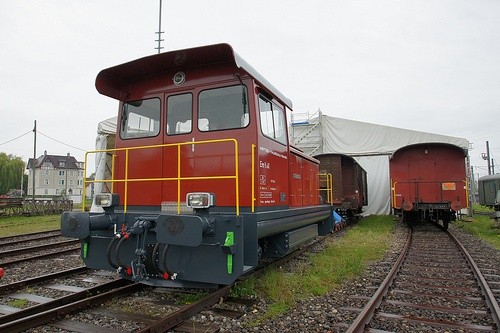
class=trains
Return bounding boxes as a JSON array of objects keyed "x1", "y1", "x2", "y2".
[
  {"x1": 388, "y1": 142, "x2": 469, "y2": 230},
  {"x1": 60, "y1": 42, "x2": 368, "y2": 292}
]
